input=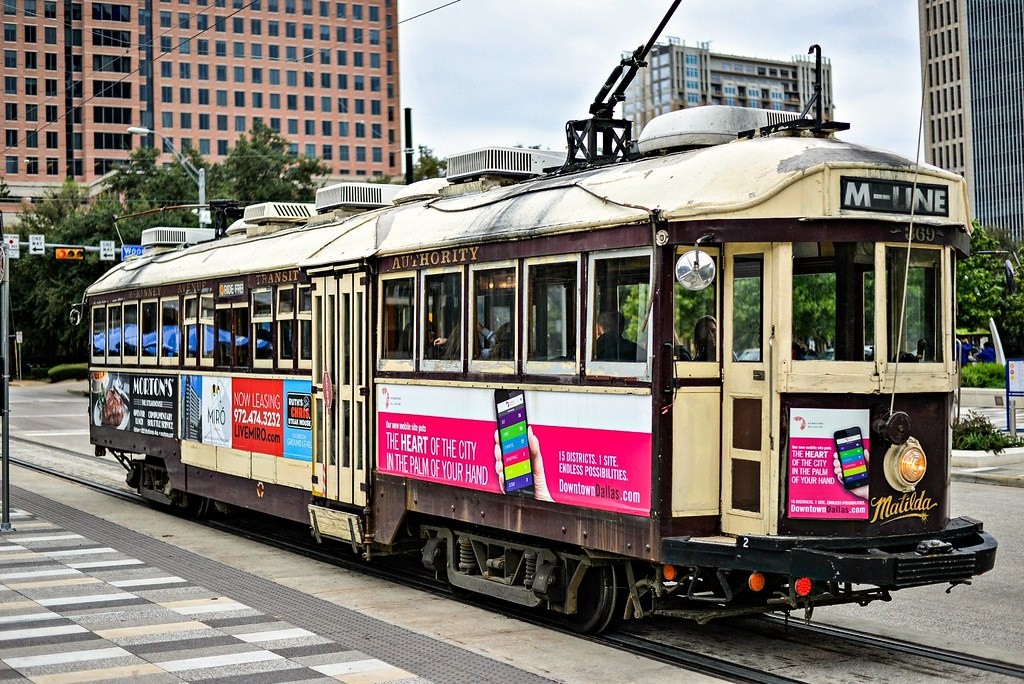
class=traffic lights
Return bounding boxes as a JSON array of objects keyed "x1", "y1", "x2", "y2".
[{"x1": 53, "y1": 247, "x2": 85, "y2": 261}]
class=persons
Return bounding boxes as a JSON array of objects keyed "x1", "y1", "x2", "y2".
[
  {"x1": 486, "y1": 322, "x2": 515, "y2": 360},
  {"x1": 595, "y1": 310, "x2": 647, "y2": 362},
  {"x1": 956, "y1": 337, "x2": 962, "y2": 359},
  {"x1": 394, "y1": 319, "x2": 449, "y2": 360},
  {"x1": 692, "y1": 315, "x2": 739, "y2": 363},
  {"x1": 961, "y1": 338, "x2": 972, "y2": 368},
  {"x1": 976, "y1": 342, "x2": 995, "y2": 362},
  {"x1": 791, "y1": 340, "x2": 809, "y2": 360},
  {"x1": 674, "y1": 330, "x2": 692, "y2": 361},
  {"x1": 440, "y1": 319, "x2": 498, "y2": 361}
]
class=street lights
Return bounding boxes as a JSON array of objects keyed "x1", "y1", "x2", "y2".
[{"x1": 127, "y1": 127, "x2": 209, "y2": 229}]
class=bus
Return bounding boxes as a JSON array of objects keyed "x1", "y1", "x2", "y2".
[{"x1": 68, "y1": 105, "x2": 1001, "y2": 640}]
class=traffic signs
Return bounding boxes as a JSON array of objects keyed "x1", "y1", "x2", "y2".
[
  {"x1": 99, "y1": 240, "x2": 116, "y2": 261},
  {"x1": 29, "y1": 234, "x2": 46, "y2": 255}
]
